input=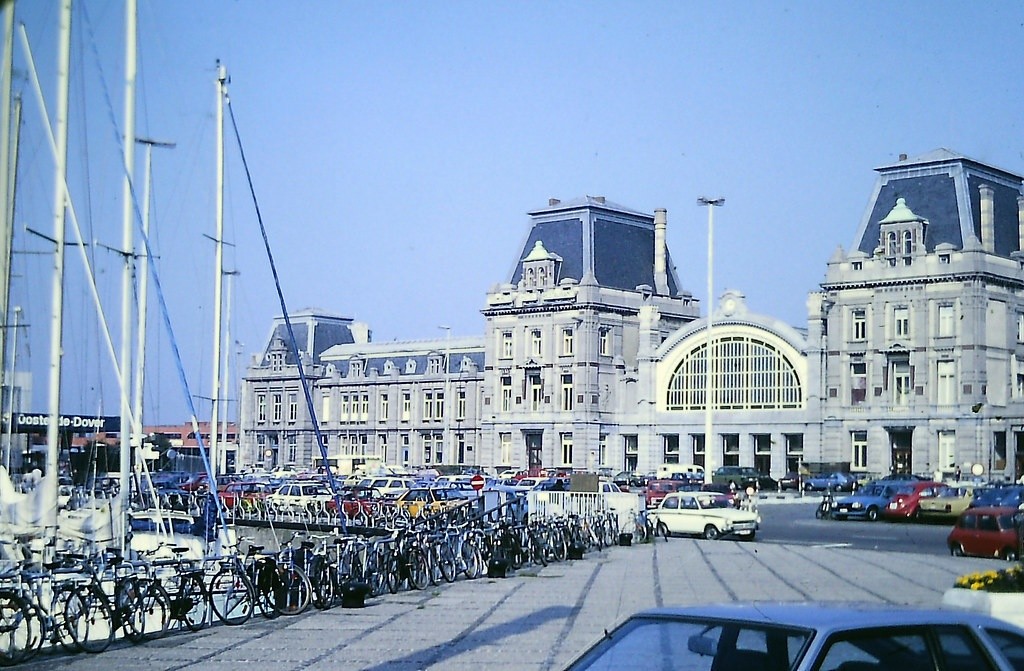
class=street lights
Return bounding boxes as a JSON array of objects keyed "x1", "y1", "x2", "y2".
[
  {"x1": 695, "y1": 197, "x2": 726, "y2": 486},
  {"x1": 6, "y1": 306, "x2": 22, "y2": 475},
  {"x1": 437, "y1": 324, "x2": 452, "y2": 465}
]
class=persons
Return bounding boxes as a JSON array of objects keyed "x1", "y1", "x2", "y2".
[
  {"x1": 726, "y1": 478, "x2": 740, "y2": 510},
  {"x1": 954, "y1": 464, "x2": 961, "y2": 483},
  {"x1": 550, "y1": 479, "x2": 565, "y2": 491}
]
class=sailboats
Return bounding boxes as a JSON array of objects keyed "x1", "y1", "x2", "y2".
[{"x1": 1, "y1": 0, "x2": 256, "y2": 564}]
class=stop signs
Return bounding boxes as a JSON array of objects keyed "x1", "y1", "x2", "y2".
[{"x1": 471, "y1": 476, "x2": 486, "y2": 491}]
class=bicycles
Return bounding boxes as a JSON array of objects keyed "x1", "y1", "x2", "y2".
[{"x1": 0, "y1": 500, "x2": 672, "y2": 667}]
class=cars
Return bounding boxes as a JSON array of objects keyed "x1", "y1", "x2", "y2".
[
  {"x1": 646, "y1": 493, "x2": 761, "y2": 540},
  {"x1": 947, "y1": 505, "x2": 1024, "y2": 562},
  {"x1": 58, "y1": 462, "x2": 706, "y2": 517},
  {"x1": 712, "y1": 463, "x2": 1024, "y2": 530},
  {"x1": 557, "y1": 601, "x2": 1024, "y2": 671}
]
